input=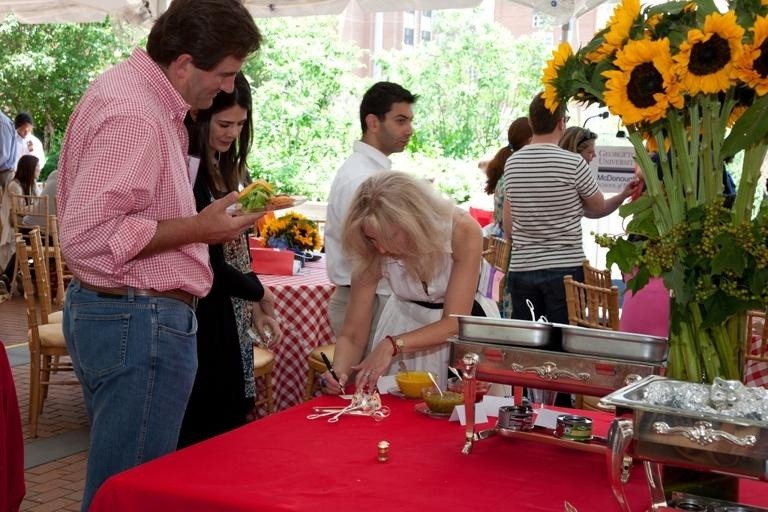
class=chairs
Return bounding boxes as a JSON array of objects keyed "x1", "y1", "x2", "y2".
[
  {"x1": 745, "y1": 308, "x2": 768, "y2": 361},
  {"x1": 15, "y1": 233, "x2": 81, "y2": 438},
  {"x1": 481, "y1": 246, "x2": 496, "y2": 265},
  {"x1": 50, "y1": 215, "x2": 76, "y2": 374},
  {"x1": 487, "y1": 235, "x2": 510, "y2": 271},
  {"x1": 305, "y1": 345, "x2": 335, "y2": 400},
  {"x1": 254, "y1": 345, "x2": 275, "y2": 414},
  {"x1": 8, "y1": 192, "x2": 53, "y2": 300},
  {"x1": 582, "y1": 260, "x2": 612, "y2": 289},
  {"x1": 563, "y1": 275, "x2": 619, "y2": 331},
  {"x1": 28, "y1": 225, "x2": 63, "y2": 324}
]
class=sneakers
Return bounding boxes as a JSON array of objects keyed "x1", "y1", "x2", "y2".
[
  {"x1": 0, "y1": 274, "x2": 10, "y2": 295},
  {"x1": 16, "y1": 272, "x2": 24, "y2": 296}
]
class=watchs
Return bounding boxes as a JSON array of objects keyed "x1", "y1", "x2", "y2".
[{"x1": 392, "y1": 337, "x2": 404, "y2": 358}]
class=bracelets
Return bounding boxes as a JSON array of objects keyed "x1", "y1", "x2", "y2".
[{"x1": 384, "y1": 335, "x2": 396, "y2": 356}]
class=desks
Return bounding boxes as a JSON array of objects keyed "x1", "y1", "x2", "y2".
[
  {"x1": 89, "y1": 385, "x2": 767, "y2": 511},
  {"x1": 256, "y1": 253, "x2": 335, "y2": 414}
]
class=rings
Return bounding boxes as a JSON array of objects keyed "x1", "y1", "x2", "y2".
[{"x1": 320, "y1": 379, "x2": 325, "y2": 388}]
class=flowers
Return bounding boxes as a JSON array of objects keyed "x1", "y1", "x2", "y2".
[
  {"x1": 542, "y1": 1, "x2": 768, "y2": 383},
  {"x1": 260, "y1": 211, "x2": 322, "y2": 252}
]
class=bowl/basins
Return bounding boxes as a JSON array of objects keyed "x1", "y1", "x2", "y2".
[
  {"x1": 449, "y1": 378, "x2": 492, "y2": 402},
  {"x1": 422, "y1": 387, "x2": 464, "y2": 412},
  {"x1": 397, "y1": 372, "x2": 439, "y2": 398}
]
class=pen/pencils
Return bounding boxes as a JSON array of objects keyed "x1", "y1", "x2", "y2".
[{"x1": 320, "y1": 352, "x2": 346, "y2": 395}]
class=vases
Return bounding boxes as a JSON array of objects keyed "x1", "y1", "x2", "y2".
[{"x1": 290, "y1": 246, "x2": 306, "y2": 269}]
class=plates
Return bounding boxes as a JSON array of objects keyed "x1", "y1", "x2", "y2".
[
  {"x1": 227, "y1": 195, "x2": 307, "y2": 215},
  {"x1": 415, "y1": 402, "x2": 452, "y2": 416}
]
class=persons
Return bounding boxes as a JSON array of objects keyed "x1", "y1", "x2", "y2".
[
  {"x1": 503, "y1": 91, "x2": 606, "y2": 402},
  {"x1": 318, "y1": 173, "x2": 485, "y2": 397},
  {"x1": 56, "y1": 0, "x2": 262, "y2": 512},
  {"x1": 0, "y1": 107, "x2": 55, "y2": 299},
  {"x1": 187, "y1": 71, "x2": 283, "y2": 449},
  {"x1": 484, "y1": 117, "x2": 533, "y2": 318},
  {"x1": 326, "y1": 80, "x2": 420, "y2": 358},
  {"x1": 560, "y1": 128, "x2": 639, "y2": 219},
  {"x1": 616, "y1": 133, "x2": 737, "y2": 336}
]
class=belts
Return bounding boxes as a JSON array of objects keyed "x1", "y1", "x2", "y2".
[{"x1": 73, "y1": 276, "x2": 199, "y2": 312}]
